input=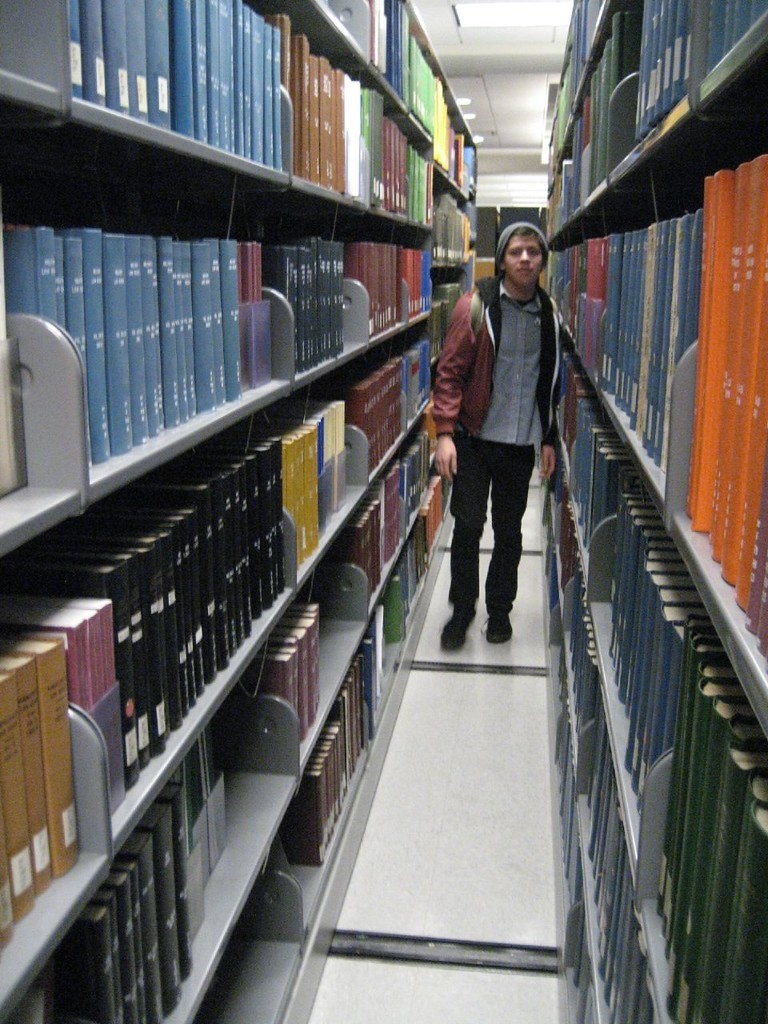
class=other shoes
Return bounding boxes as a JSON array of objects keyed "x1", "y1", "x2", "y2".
[
  {"x1": 486, "y1": 608, "x2": 512, "y2": 643},
  {"x1": 441, "y1": 602, "x2": 476, "y2": 650}
]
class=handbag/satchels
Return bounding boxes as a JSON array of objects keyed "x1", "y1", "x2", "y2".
[{"x1": 430, "y1": 286, "x2": 482, "y2": 395}]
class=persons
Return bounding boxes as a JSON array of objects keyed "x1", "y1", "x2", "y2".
[{"x1": 430, "y1": 222, "x2": 563, "y2": 652}]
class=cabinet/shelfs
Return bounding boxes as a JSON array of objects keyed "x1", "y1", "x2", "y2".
[
  {"x1": 517, "y1": 0, "x2": 768, "y2": 1024},
  {"x1": 0, "y1": 0, "x2": 479, "y2": 1024}
]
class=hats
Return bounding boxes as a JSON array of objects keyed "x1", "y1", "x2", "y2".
[{"x1": 495, "y1": 221, "x2": 549, "y2": 272}]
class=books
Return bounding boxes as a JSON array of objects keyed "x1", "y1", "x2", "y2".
[
  {"x1": 536, "y1": 0, "x2": 768, "y2": 1024},
  {"x1": 0, "y1": 0, "x2": 476, "y2": 1024}
]
